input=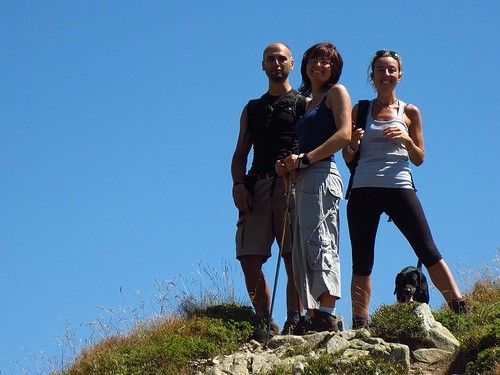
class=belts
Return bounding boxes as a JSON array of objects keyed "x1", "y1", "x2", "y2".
[{"x1": 256, "y1": 171, "x2": 280, "y2": 181}]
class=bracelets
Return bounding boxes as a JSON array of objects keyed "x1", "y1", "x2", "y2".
[
  {"x1": 232, "y1": 181, "x2": 245, "y2": 186},
  {"x1": 299, "y1": 153, "x2": 310, "y2": 169},
  {"x1": 406, "y1": 140, "x2": 415, "y2": 152}
]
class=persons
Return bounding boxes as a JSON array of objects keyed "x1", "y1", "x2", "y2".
[
  {"x1": 275, "y1": 42, "x2": 352, "y2": 335},
  {"x1": 342, "y1": 49, "x2": 471, "y2": 332},
  {"x1": 231, "y1": 43, "x2": 313, "y2": 343}
]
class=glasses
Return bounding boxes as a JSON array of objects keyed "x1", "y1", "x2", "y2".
[
  {"x1": 376, "y1": 49, "x2": 397, "y2": 58},
  {"x1": 308, "y1": 58, "x2": 333, "y2": 68}
]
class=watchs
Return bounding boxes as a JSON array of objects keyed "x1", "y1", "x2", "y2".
[{"x1": 347, "y1": 145, "x2": 358, "y2": 154}]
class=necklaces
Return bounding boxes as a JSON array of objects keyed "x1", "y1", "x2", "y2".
[{"x1": 376, "y1": 98, "x2": 397, "y2": 108}]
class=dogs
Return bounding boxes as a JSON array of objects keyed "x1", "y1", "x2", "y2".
[{"x1": 392, "y1": 256, "x2": 430, "y2": 306}]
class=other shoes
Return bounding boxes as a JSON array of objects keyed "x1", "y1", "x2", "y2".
[
  {"x1": 294, "y1": 309, "x2": 338, "y2": 335},
  {"x1": 455, "y1": 302, "x2": 469, "y2": 313},
  {"x1": 282, "y1": 320, "x2": 297, "y2": 336},
  {"x1": 268, "y1": 324, "x2": 279, "y2": 337}
]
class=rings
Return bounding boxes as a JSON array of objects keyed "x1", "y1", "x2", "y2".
[{"x1": 287, "y1": 164, "x2": 291, "y2": 168}]
